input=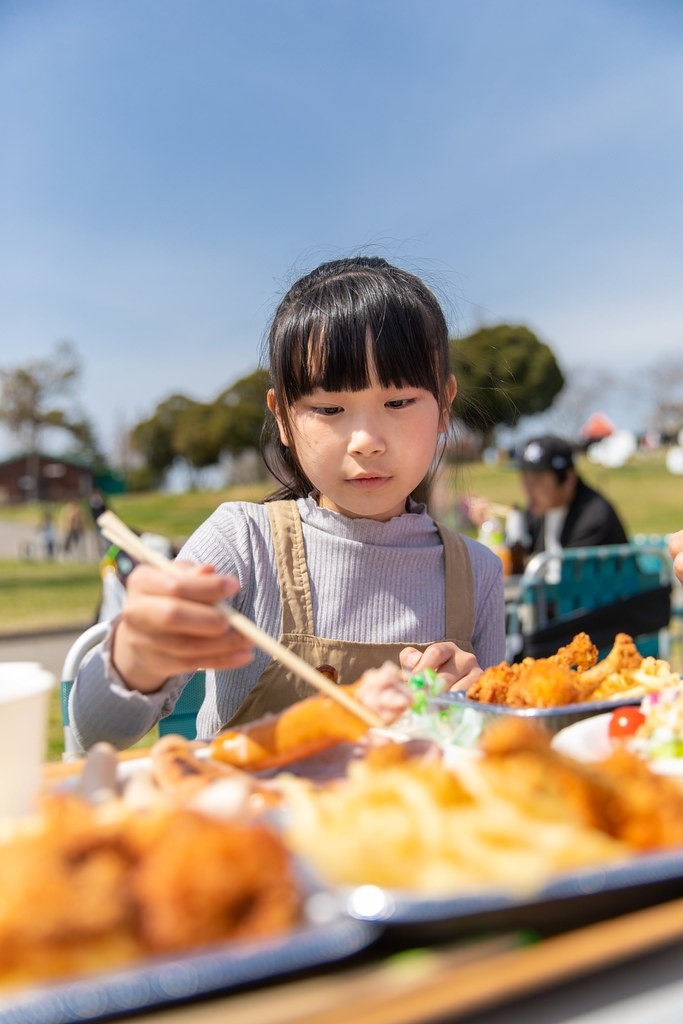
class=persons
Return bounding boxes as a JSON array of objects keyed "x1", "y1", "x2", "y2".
[
  {"x1": 68, "y1": 257, "x2": 505, "y2": 756},
  {"x1": 505, "y1": 435, "x2": 629, "y2": 574}
]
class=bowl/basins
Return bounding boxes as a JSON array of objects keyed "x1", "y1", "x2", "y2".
[{"x1": 425, "y1": 690, "x2": 650, "y2": 752}]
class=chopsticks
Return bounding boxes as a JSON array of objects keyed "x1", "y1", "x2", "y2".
[{"x1": 95, "y1": 508, "x2": 384, "y2": 730}]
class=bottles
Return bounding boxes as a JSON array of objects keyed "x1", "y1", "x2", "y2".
[{"x1": 490, "y1": 534, "x2": 512, "y2": 578}]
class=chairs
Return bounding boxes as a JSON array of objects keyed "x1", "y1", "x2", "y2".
[{"x1": 518, "y1": 544, "x2": 673, "y2": 664}]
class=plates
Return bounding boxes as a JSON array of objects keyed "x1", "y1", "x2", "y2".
[
  {"x1": 551, "y1": 710, "x2": 683, "y2": 776},
  {"x1": 329, "y1": 850, "x2": 683, "y2": 939},
  {"x1": 0, "y1": 897, "x2": 387, "y2": 1024}
]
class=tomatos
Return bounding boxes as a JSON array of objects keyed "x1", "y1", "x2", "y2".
[{"x1": 608, "y1": 709, "x2": 646, "y2": 739}]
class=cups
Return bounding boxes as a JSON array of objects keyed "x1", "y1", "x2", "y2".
[{"x1": 0, "y1": 661, "x2": 52, "y2": 837}]
